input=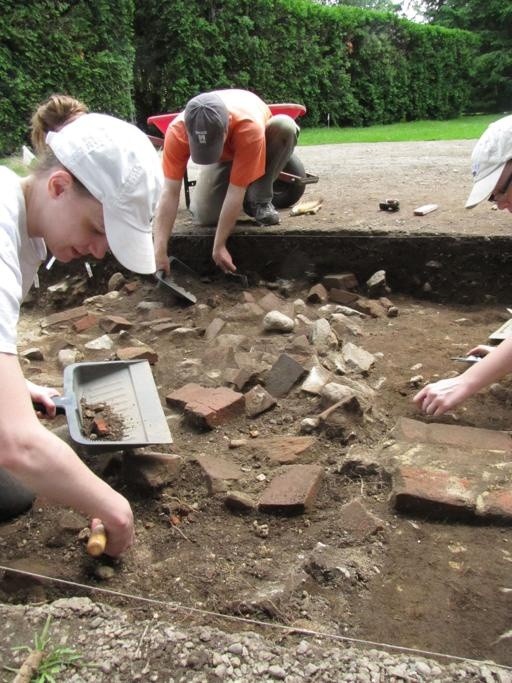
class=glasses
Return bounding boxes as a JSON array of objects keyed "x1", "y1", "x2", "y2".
[{"x1": 488, "y1": 172, "x2": 512, "y2": 204}]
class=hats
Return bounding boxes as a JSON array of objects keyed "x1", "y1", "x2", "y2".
[
  {"x1": 184, "y1": 93, "x2": 228, "y2": 165},
  {"x1": 464, "y1": 114, "x2": 512, "y2": 210},
  {"x1": 45, "y1": 112, "x2": 165, "y2": 275}
]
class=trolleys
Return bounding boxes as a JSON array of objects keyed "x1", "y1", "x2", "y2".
[{"x1": 147, "y1": 103, "x2": 319, "y2": 209}]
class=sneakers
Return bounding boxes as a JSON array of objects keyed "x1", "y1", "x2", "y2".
[{"x1": 243, "y1": 202, "x2": 279, "y2": 225}]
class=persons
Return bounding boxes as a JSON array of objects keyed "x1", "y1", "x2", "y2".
[
  {"x1": 413, "y1": 115, "x2": 512, "y2": 416},
  {"x1": 153, "y1": 86, "x2": 301, "y2": 276},
  {"x1": 0, "y1": 93, "x2": 165, "y2": 557}
]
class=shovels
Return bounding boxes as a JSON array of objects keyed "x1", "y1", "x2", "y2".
[
  {"x1": 156, "y1": 255, "x2": 200, "y2": 303},
  {"x1": 34, "y1": 360, "x2": 173, "y2": 448}
]
